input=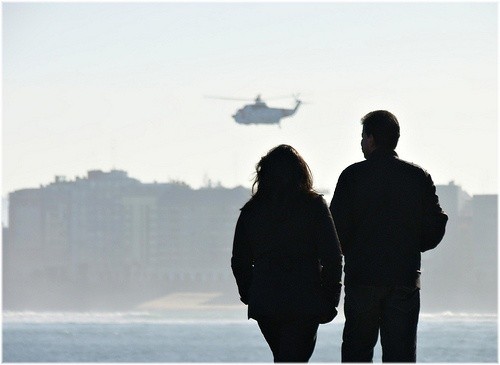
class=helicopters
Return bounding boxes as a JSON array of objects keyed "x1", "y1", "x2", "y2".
[{"x1": 215, "y1": 94, "x2": 303, "y2": 126}]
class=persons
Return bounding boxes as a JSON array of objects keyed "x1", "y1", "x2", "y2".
[
  {"x1": 229, "y1": 143, "x2": 344, "y2": 363},
  {"x1": 328, "y1": 108, "x2": 449, "y2": 363}
]
